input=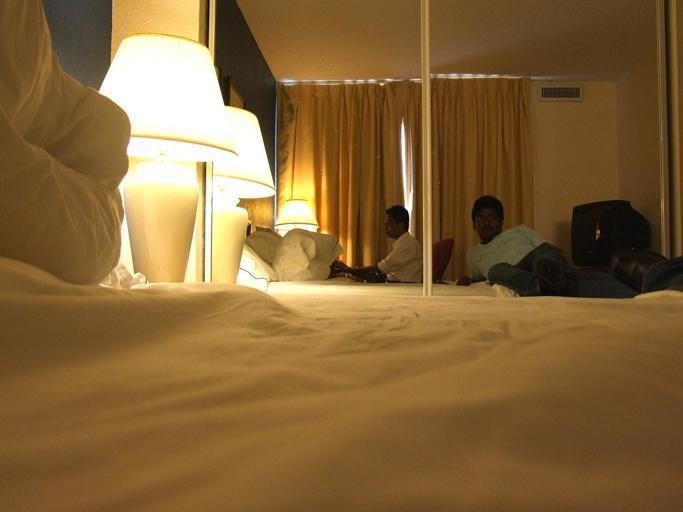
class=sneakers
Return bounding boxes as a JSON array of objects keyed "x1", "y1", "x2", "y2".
[{"x1": 535, "y1": 258, "x2": 576, "y2": 294}]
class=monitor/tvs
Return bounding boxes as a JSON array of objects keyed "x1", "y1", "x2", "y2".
[{"x1": 570, "y1": 199, "x2": 650, "y2": 267}]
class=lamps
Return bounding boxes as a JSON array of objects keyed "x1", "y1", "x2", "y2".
[
  {"x1": 274, "y1": 199, "x2": 319, "y2": 236},
  {"x1": 97, "y1": 33, "x2": 239, "y2": 283},
  {"x1": 212, "y1": 106, "x2": 276, "y2": 284}
]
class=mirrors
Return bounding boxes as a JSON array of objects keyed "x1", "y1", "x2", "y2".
[
  {"x1": 426, "y1": 0, "x2": 666, "y2": 286},
  {"x1": 208, "y1": 0, "x2": 426, "y2": 286}
]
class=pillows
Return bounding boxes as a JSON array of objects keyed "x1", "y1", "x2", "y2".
[
  {"x1": 432, "y1": 238, "x2": 454, "y2": 283},
  {"x1": 0, "y1": 0, "x2": 130, "y2": 285},
  {"x1": 271, "y1": 228, "x2": 339, "y2": 282}
]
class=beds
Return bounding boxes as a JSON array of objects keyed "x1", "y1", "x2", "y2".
[{"x1": 0, "y1": 258, "x2": 683, "y2": 512}]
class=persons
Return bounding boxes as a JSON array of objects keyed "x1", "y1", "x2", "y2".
[
  {"x1": 332, "y1": 205, "x2": 423, "y2": 282},
  {"x1": 456, "y1": 194, "x2": 635, "y2": 298}
]
